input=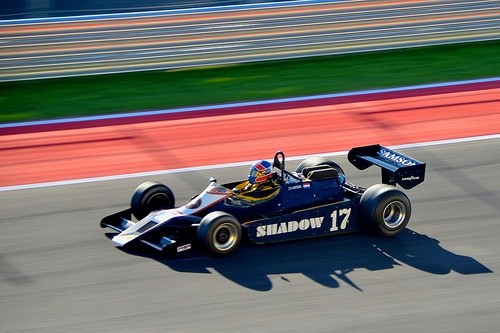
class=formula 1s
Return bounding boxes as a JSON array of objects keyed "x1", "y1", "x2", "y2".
[{"x1": 98, "y1": 143, "x2": 426, "y2": 261}]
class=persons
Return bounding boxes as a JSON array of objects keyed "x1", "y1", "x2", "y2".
[{"x1": 225, "y1": 160, "x2": 279, "y2": 205}]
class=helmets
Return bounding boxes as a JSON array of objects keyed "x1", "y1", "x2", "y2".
[{"x1": 248, "y1": 160, "x2": 273, "y2": 184}]
